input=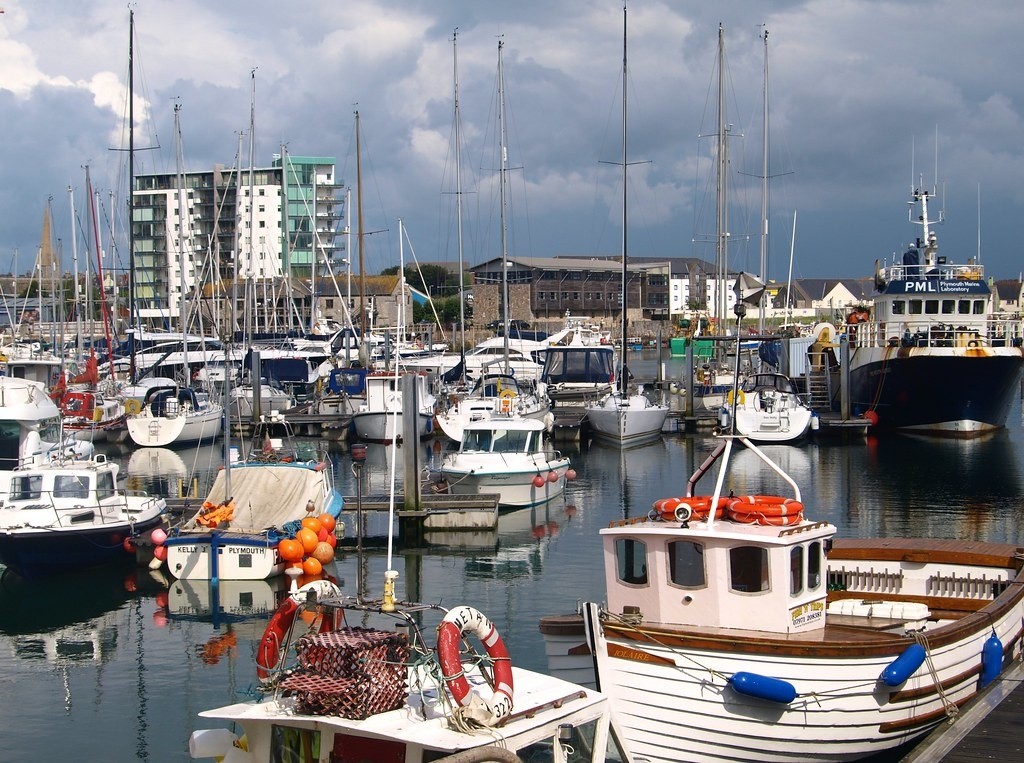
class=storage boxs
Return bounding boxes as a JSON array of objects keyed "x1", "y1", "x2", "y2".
[{"x1": 276, "y1": 626, "x2": 411, "y2": 719}]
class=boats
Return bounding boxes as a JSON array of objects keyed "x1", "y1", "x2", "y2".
[
  {"x1": 840, "y1": 118, "x2": 1023, "y2": 438},
  {"x1": 538, "y1": 300, "x2": 1022, "y2": 763}
]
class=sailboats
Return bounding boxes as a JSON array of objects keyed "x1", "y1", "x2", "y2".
[
  {"x1": 188, "y1": 218, "x2": 640, "y2": 763},
  {"x1": 0, "y1": 135, "x2": 347, "y2": 637},
  {"x1": 585, "y1": 0, "x2": 671, "y2": 451},
  {"x1": 441, "y1": 26, "x2": 572, "y2": 510},
  {"x1": 662, "y1": 24, "x2": 837, "y2": 447},
  {"x1": 0, "y1": 0, "x2": 618, "y2": 443}
]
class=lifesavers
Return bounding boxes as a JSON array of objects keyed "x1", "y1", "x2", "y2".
[
  {"x1": 727, "y1": 388, "x2": 746, "y2": 406},
  {"x1": 498, "y1": 388, "x2": 518, "y2": 399},
  {"x1": 967, "y1": 339, "x2": 978, "y2": 348},
  {"x1": 125, "y1": 398, "x2": 141, "y2": 416},
  {"x1": 654, "y1": 494, "x2": 805, "y2": 527},
  {"x1": 257, "y1": 579, "x2": 345, "y2": 687},
  {"x1": 436, "y1": 601, "x2": 515, "y2": 727}
]
